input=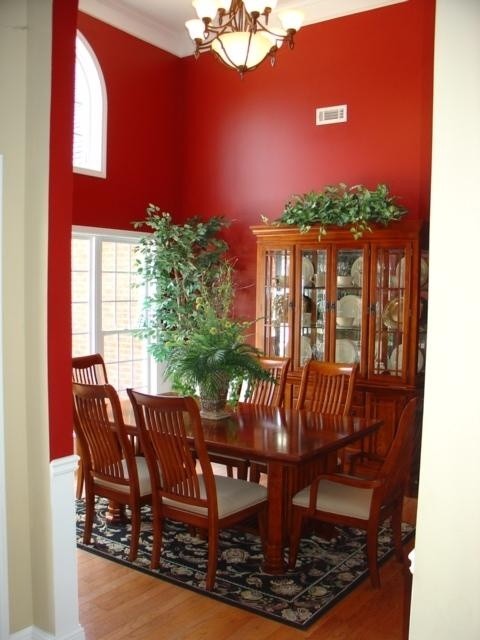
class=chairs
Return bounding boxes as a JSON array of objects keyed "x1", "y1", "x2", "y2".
[
  {"x1": 285, "y1": 394, "x2": 423, "y2": 590},
  {"x1": 68, "y1": 350, "x2": 124, "y2": 498},
  {"x1": 248, "y1": 357, "x2": 362, "y2": 485},
  {"x1": 187, "y1": 353, "x2": 291, "y2": 475},
  {"x1": 69, "y1": 377, "x2": 164, "y2": 558},
  {"x1": 126, "y1": 382, "x2": 267, "y2": 590}
]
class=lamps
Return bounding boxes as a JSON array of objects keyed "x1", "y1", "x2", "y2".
[{"x1": 184, "y1": 0, "x2": 309, "y2": 79}]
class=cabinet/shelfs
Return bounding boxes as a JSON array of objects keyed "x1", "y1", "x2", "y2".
[{"x1": 247, "y1": 218, "x2": 430, "y2": 498}]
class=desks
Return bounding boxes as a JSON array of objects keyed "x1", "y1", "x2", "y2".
[{"x1": 76, "y1": 392, "x2": 382, "y2": 575}]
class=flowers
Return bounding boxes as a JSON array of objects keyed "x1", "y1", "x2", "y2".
[{"x1": 153, "y1": 295, "x2": 282, "y2": 411}]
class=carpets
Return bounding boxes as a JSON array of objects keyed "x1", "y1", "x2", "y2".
[{"x1": 70, "y1": 487, "x2": 417, "y2": 633}]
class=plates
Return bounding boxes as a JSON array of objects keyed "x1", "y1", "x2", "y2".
[{"x1": 276, "y1": 253, "x2": 429, "y2": 376}]
